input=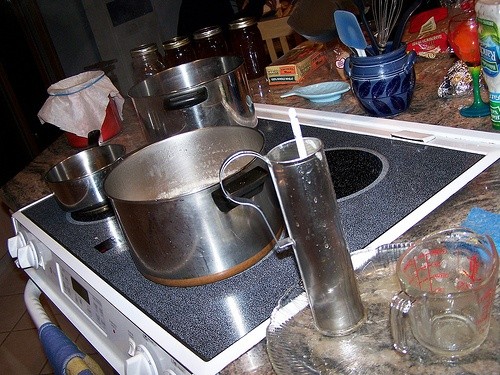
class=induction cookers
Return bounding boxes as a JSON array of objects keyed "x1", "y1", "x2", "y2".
[{"x1": 20, "y1": 119, "x2": 486, "y2": 362}]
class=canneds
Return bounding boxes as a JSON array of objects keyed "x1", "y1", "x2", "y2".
[{"x1": 130, "y1": 16, "x2": 267, "y2": 84}]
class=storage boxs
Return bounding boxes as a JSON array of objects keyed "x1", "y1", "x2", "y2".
[{"x1": 265, "y1": 39, "x2": 329, "y2": 86}]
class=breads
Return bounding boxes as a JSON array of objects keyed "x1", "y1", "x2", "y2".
[{"x1": 334, "y1": 41, "x2": 352, "y2": 80}]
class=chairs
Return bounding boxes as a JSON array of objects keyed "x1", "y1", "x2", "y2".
[{"x1": 257, "y1": 13, "x2": 307, "y2": 63}]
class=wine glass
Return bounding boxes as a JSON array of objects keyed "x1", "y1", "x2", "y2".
[{"x1": 448, "y1": 6, "x2": 492, "y2": 118}]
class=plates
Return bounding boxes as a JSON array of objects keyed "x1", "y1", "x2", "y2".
[
  {"x1": 266, "y1": 242, "x2": 500, "y2": 375},
  {"x1": 278, "y1": 80, "x2": 351, "y2": 105}
]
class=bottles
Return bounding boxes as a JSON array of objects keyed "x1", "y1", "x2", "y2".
[{"x1": 474, "y1": 0, "x2": 500, "y2": 130}]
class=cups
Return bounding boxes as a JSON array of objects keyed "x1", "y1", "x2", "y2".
[
  {"x1": 217, "y1": 138, "x2": 367, "y2": 338},
  {"x1": 389, "y1": 228, "x2": 499, "y2": 358}
]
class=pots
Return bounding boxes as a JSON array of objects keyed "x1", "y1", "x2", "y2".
[
  {"x1": 128, "y1": 56, "x2": 258, "y2": 144},
  {"x1": 44, "y1": 144, "x2": 126, "y2": 212},
  {"x1": 101, "y1": 127, "x2": 284, "y2": 287}
]
